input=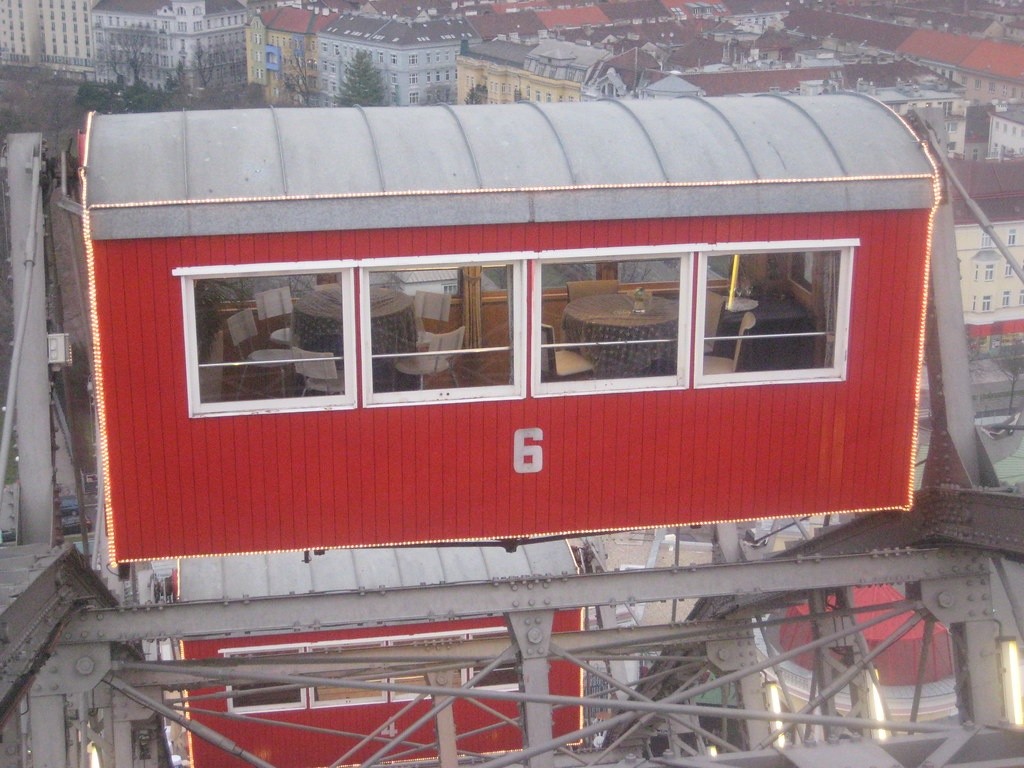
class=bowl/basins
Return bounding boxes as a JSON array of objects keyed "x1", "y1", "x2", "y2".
[{"x1": 623, "y1": 295, "x2": 662, "y2": 314}]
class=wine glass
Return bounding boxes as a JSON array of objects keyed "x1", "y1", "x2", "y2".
[{"x1": 736, "y1": 286, "x2": 753, "y2": 298}]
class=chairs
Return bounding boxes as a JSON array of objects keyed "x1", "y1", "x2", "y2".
[
  {"x1": 252, "y1": 286, "x2": 293, "y2": 349},
  {"x1": 703, "y1": 311, "x2": 757, "y2": 375},
  {"x1": 416, "y1": 289, "x2": 452, "y2": 350},
  {"x1": 391, "y1": 324, "x2": 466, "y2": 392},
  {"x1": 227, "y1": 308, "x2": 293, "y2": 401},
  {"x1": 291, "y1": 347, "x2": 345, "y2": 396},
  {"x1": 565, "y1": 279, "x2": 620, "y2": 301},
  {"x1": 704, "y1": 290, "x2": 726, "y2": 356},
  {"x1": 541, "y1": 323, "x2": 595, "y2": 382}
]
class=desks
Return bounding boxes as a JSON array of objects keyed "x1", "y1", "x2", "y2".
[
  {"x1": 560, "y1": 294, "x2": 679, "y2": 377},
  {"x1": 721, "y1": 293, "x2": 806, "y2": 355},
  {"x1": 289, "y1": 283, "x2": 419, "y2": 383}
]
